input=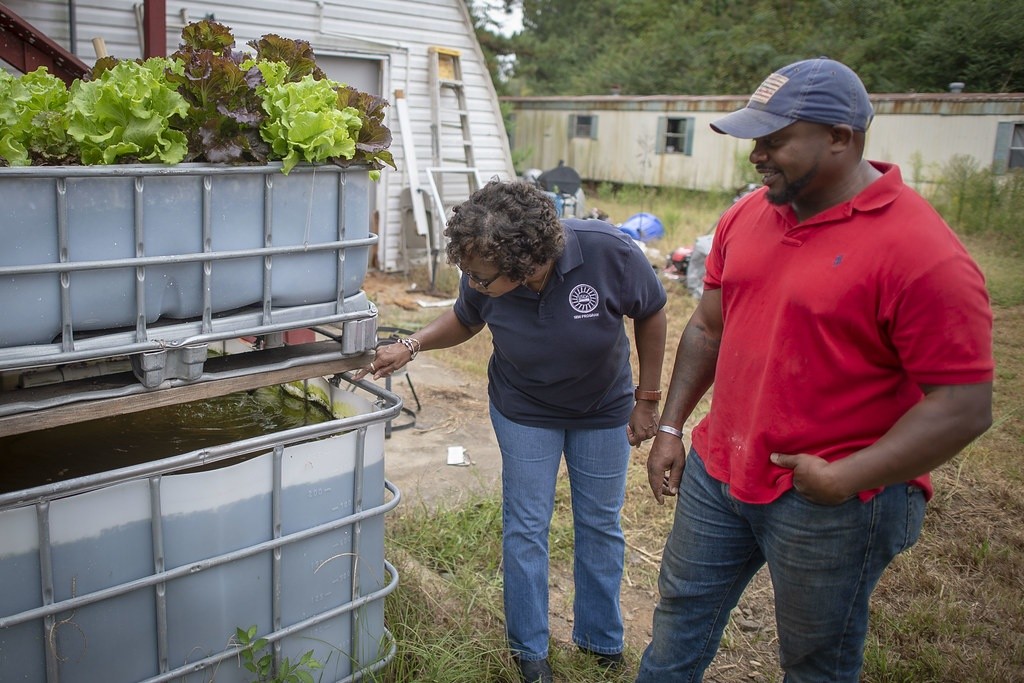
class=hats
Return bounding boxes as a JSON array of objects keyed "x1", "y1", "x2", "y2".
[{"x1": 710, "y1": 56, "x2": 874, "y2": 139}]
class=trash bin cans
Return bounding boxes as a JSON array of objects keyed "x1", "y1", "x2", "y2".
[{"x1": 618, "y1": 213, "x2": 666, "y2": 243}]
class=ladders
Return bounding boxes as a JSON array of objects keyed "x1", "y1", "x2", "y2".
[{"x1": 427, "y1": 45, "x2": 480, "y2": 264}]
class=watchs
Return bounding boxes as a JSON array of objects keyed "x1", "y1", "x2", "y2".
[{"x1": 634, "y1": 385, "x2": 662, "y2": 402}]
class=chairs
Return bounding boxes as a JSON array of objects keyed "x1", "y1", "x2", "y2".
[{"x1": 398, "y1": 187, "x2": 437, "y2": 278}]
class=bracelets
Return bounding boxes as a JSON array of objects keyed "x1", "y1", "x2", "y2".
[
  {"x1": 398, "y1": 338, "x2": 420, "y2": 361},
  {"x1": 657, "y1": 425, "x2": 683, "y2": 439}
]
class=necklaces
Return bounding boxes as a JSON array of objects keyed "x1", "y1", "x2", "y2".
[{"x1": 537, "y1": 257, "x2": 552, "y2": 294}]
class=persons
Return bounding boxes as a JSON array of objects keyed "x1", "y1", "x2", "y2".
[
  {"x1": 350, "y1": 175, "x2": 667, "y2": 683},
  {"x1": 633, "y1": 56, "x2": 996, "y2": 683}
]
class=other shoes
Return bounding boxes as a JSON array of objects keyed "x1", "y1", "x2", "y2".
[
  {"x1": 511, "y1": 654, "x2": 553, "y2": 683},
  {"x1": 577, "y1": 643, "x2": 630, "y2": 682}
]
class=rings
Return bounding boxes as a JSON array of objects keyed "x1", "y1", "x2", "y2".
[{"x1": 371, "y1": 363, "x2": 375, "y2": 370}]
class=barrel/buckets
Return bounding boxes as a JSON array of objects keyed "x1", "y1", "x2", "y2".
[{"x1": 619, "y1": 212, "x2": 664, "y2": 241}]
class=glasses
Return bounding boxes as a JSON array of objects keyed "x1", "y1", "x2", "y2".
[{"x1": 455, "y1": 258, "x2": 504, "y2": 289}]
